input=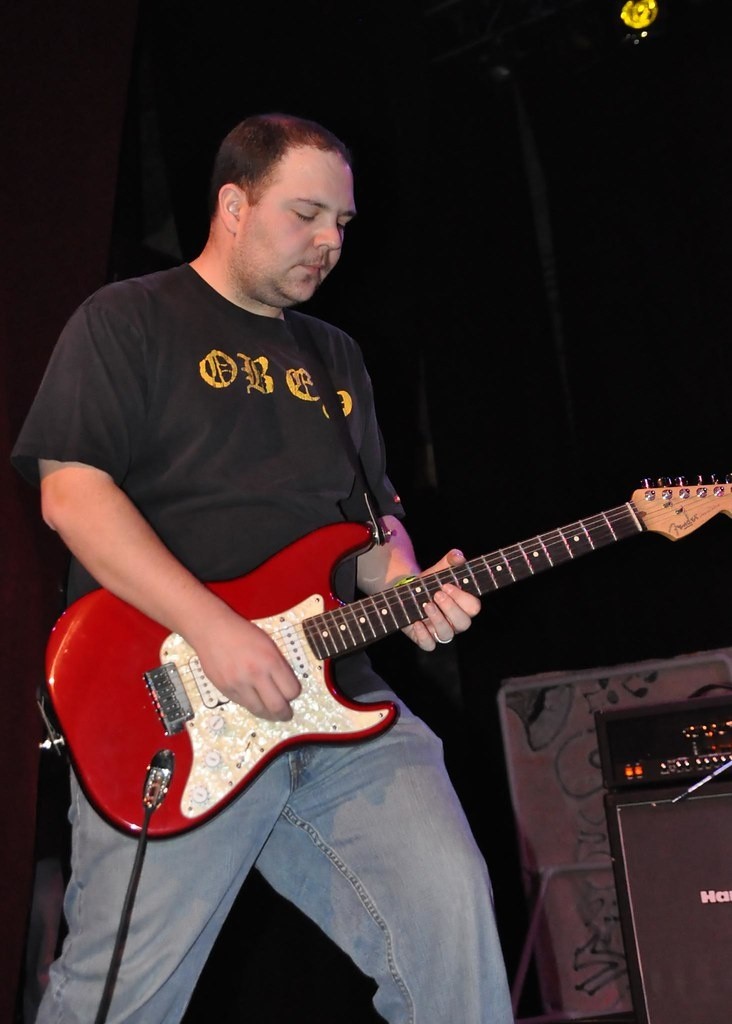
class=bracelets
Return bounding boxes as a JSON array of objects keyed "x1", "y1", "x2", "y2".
[{"x1": 394, "y1": 574, "x2": 416, "y2": 588}]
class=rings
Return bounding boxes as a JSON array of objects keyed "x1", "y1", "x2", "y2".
[{"x1": 435, "y1": 632, "x2": 454, "y2": 644}]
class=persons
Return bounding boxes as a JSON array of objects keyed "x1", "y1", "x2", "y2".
[{"x1": 12, "y1": 114, "x2": 518, "y2": 1024}]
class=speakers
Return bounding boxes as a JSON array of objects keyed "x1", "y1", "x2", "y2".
[{"x1": 603, "y1": 780, "x2": 732, "y2": 1024}]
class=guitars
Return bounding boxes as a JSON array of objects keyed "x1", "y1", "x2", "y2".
[{"x1": 42, "y1": 475, "x2": 732, "y2": 843}]
